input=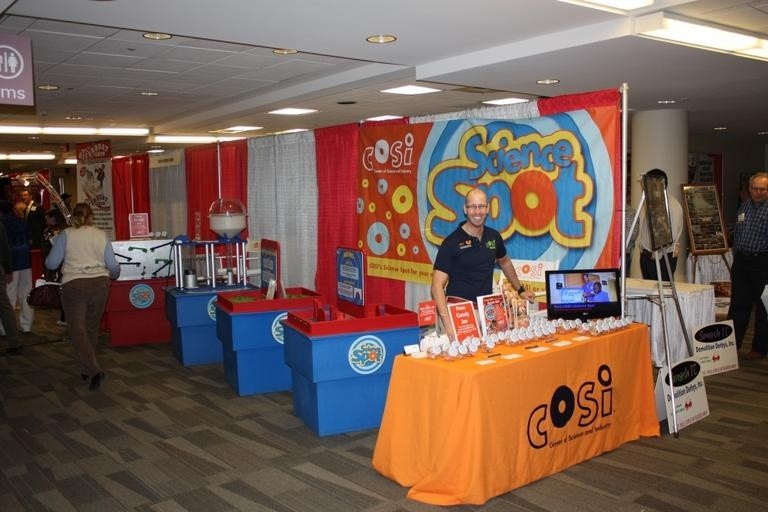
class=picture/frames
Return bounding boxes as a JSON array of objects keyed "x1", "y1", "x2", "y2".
[
  {"x1": 681, "y1": 181, "x2": 728, "y2": 254},
  {"x1": 642, "y1": 170, "x2": 671, "y2": 251}
]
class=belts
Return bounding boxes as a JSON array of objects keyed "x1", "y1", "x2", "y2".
[
  {"x1": 447, "y1": 297, "x2": 478, "y2": 309},
  {"x1": 740, "y1": 251, "x2": 765, "y2": 257}
]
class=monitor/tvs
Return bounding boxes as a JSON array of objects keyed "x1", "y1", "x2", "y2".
[{"x1": 545, "y1": 266, "x2": 621, "y2": 323}]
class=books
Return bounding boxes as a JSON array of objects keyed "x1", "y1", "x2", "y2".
[{"x1": 436, "y1": 284, "x2": 539, "y2": 341}]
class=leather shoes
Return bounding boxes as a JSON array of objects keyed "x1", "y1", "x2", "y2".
[
  {"x1": 89, "y1": 367, "x2": 105, "y2": 391},
  {"x1": 744, "y1": 352, "x2": 764, "y2": 360}
]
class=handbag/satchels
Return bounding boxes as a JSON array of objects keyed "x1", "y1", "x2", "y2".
[{"x1": 26, "y1": 270, "x2": 63, "y2": 310}]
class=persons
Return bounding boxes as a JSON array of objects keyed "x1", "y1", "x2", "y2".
[
  {"x1": 623, "y1": 205, "x2": 639, "y2": 277},
  {"x1": 639, "y1": 168, "x2": 683, "y2": 282},
  {"x1": 44, "y1": 202, "x2": 122, "y2": 392},
  {"x1": 592, "y1": 282, "x2": 610, "y2": 302},
  {"x1": 0, "y1": 187, "x2": 76, "y2": 340},
  {"x1": 582, "y1": 273, "x2": 597, "y2": 302},
  {"x1": 726, "y1": 173, "x2": 767, "y2": 361},
  {"x1": 430, "y1": 187, "x2": 537, "y2": 344}
]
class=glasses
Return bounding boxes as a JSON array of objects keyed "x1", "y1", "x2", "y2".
[{"x1": 466, "y1": 204, "x2": 488, "y2": 210}]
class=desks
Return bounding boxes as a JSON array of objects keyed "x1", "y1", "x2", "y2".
[
  {"x1": 623, "y1": 278, "x2": 715, "y2": 369},
  {"x1": 370, "y1": 320, "x2": 660, "y2": 506}
]
